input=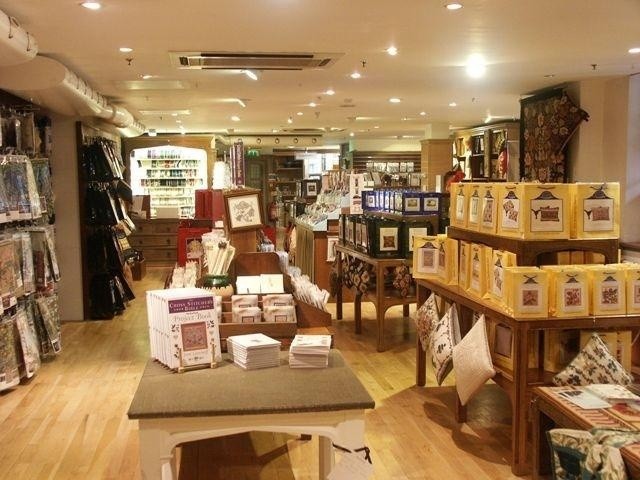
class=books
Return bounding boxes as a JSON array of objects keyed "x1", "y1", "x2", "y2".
[
  {"x1": 225, "y1": 332, "x2": 282, "y2": 371},
  {"x1": 288, "y1": 334, "x2": 332, "y2": 370},
  {"x1": 144, "y1": 286, "x2": 223, "y2": 371}
]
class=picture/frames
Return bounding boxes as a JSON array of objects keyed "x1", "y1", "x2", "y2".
[
  {"x1": 326, "y1": 236, "x2": 340, "y2": 263},
  {"x1": 338, "y1": 214, "x2": 432, "y2": 260},
  {"x1": 224, "y1": 191, "x2": 265, "y2": 234},
  {"x1": 302, "y1": 179, "x2": 320, "y2": 199}
]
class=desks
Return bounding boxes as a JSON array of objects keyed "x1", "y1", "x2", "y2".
[
  {"x1": 413, "y1": 279, "x2": 639, "y2": 479},
  {"x1": 127, "y1": 260, "x2": 375, "y2": 480},
  {"x1": 531, "y1": 383, "x2": 640, "y2": 480},
  {"x1": 446, "y1": 226, "x2": 620, "y2": 269},
  {"x1": 333, "y1": 243, "x2": 414, "y2": 352}
]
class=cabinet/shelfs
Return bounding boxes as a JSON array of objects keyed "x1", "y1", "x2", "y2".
[
  {"x1": 129, "y1": 219, "x2": 182, "y2": 267},
  {"x1": 265, "y1": 155, "x2": 304, "y2": 220},
  {"x1": 349, "y1": 151, "x2": 421, "y2": 190},
  {"x1": 451, "y1": 119, "x2": 521, "y2": 182},
  {"x1": 292, "y1": 173, "x2": 374, "y2": 302}
]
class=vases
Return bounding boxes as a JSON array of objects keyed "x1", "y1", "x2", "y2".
[{"x1": 202, "y1": 273, "x2": 234, "y2": 301}]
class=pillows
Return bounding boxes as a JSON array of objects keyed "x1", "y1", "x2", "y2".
[
  {"x1": 552, "y1": 333, "x2": 635, "y2": 387},
  {"x1": 411, "y1": 292, "x2": 498, "y2": 407}
]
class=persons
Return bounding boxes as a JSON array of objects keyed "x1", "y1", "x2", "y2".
[{"x1": 444, "y1": 170, "x2": 456, "y2": 191}]
